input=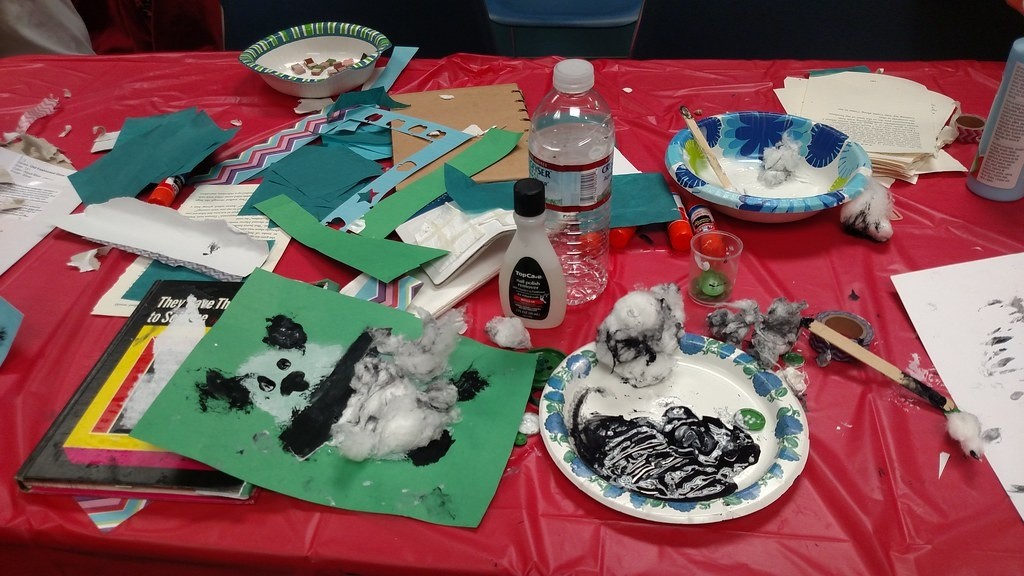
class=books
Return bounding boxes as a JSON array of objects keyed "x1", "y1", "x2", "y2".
[
  {"x1": 14, "y1": 279, "x2": 253, "y2": 500},
  {"x1": 390, "y1": 83, "x2": 538, "y2": 192}
]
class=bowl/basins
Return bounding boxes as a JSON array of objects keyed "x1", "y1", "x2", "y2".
[
  {"x1": 238, "y1": 21, "x2": 393, "y2": 100},
  {"x1": 664, "y1": 111, "x2": 872, "y2": 225}
]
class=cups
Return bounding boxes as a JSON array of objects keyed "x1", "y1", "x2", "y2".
[{"x1": 687, "y1": 230, "x2": 743, "y2": 308}]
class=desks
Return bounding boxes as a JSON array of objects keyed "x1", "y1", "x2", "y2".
[{"x1": 0, "y1": 50, "x2": 1024, "y2": 576}]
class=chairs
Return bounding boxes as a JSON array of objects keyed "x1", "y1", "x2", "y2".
[
  {"x1": 628, "y1": 0, "x2": 1024, "y2": 61},
  {"x1": 218, "y1": 0, "x2": 500, "y2": 57}
]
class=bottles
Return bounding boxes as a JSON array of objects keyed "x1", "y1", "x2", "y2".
[
  {"x1": 966, "y1": 35, "x2": 1024, "y2": 202},
  {"x1": 528, "y1": 59, "x2": 615, "y2": 306},
  {"x1": 499, "y1": 175, "x2": 568, "y2": 329}
]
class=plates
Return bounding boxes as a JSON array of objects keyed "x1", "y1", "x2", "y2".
[{"x1": 539, "y1": 332, "x2": 810, "y2": 523}]
